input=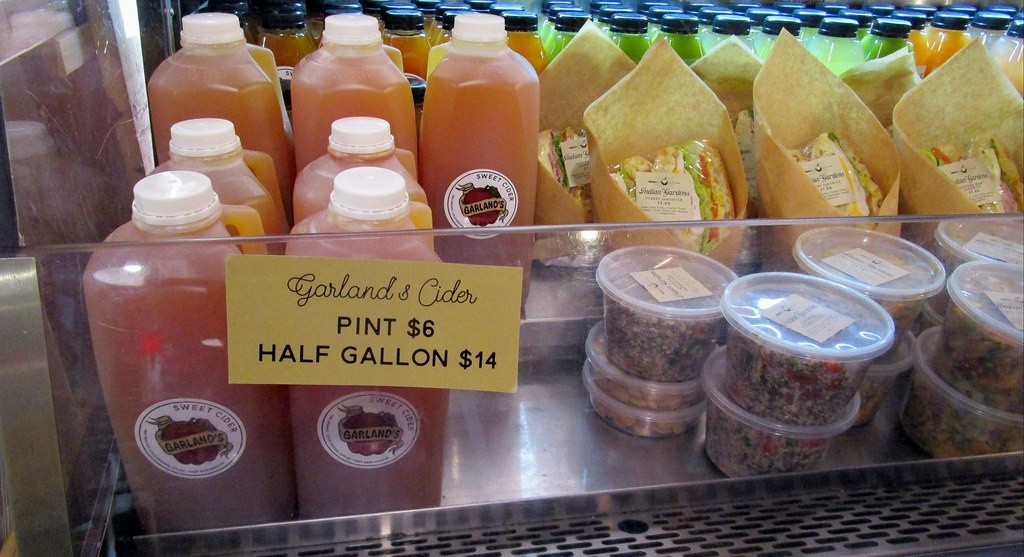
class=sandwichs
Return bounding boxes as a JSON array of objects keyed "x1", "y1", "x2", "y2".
[{"x1": 537, "y1": 106, "x2": 1024, "y2": 257}]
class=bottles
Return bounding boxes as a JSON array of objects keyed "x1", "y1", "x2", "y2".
[
  {"x1": 419, "y1": 12, "x2": 541, "y2": 309},
  {"x1": 82, "y1": 13, "x2": 289, "y2": 534},
  {"x1": 208, "y1": 0, "x2": 1024, "y2": 170},
  {"x1": 284, "y1": 14, "x2": 451, "y2": 520}
]
